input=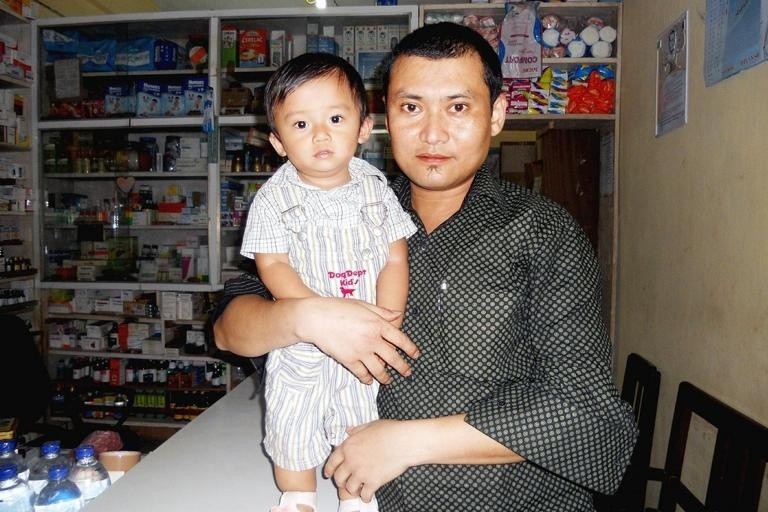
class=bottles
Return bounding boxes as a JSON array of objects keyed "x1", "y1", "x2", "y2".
[{"x1": 0, "y1": 358, "x2": 227, "y2": 512}]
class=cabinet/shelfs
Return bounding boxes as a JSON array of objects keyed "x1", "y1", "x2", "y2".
[
  {"x1": 526, "y1": 129, "x2": 599, "y2": 251},
  {"x1": 1, "y1": 0, "x2": 41, "y2": 328},
  {"x1": 41, "y1": 5, "x2": 418, "y2": 427},
  {"x1": 419, "y1": 0, "x2": 622, "y2": 113}
]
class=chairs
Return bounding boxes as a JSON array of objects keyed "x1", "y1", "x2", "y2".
[{"x1": 595, "y1": 354, "x2": 767, "y2": 512}]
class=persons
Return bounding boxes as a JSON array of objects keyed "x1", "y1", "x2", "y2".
[
  {"x1": 240, "y1": 51, "x2": 419, "y2": 510},
  {"x1": 204, "y1": 21, "x2": 640, "y2": 510}
]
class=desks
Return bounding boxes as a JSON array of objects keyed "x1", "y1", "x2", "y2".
[{"x1": 79, "y1": 367, "x2": 339, "y2": 512}]
